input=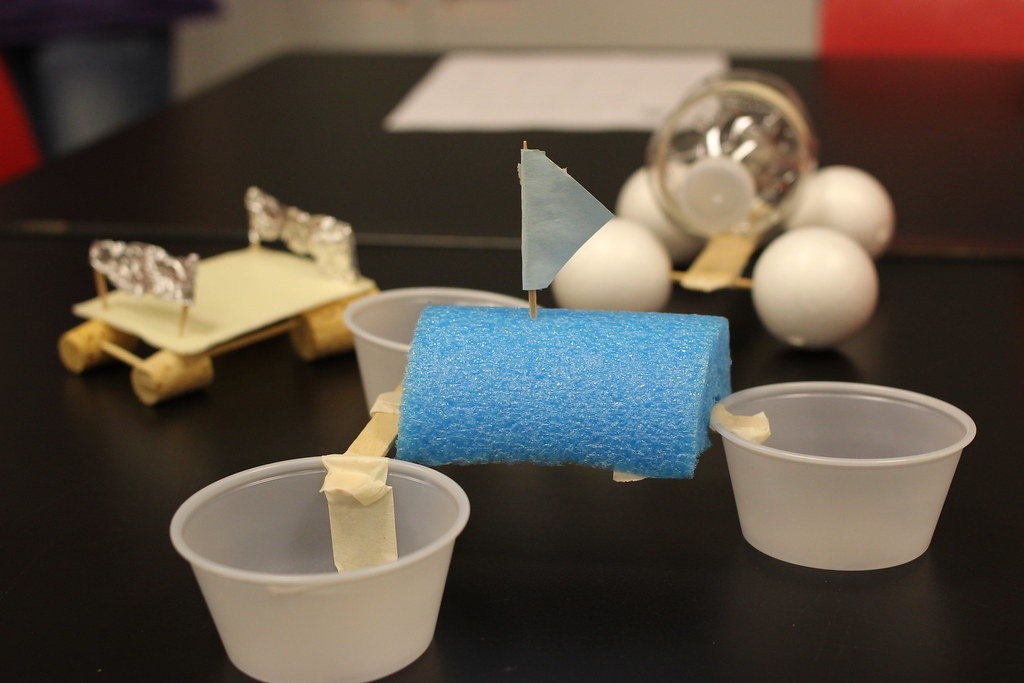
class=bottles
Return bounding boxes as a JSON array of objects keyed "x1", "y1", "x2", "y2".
[{"x1": 648, "y1": 67, "x2": 816, "y2": 252}]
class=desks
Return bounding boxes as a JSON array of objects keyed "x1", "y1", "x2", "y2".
[{"x1": 0, "y1": 49, "x2": 1024, "y2": 683}]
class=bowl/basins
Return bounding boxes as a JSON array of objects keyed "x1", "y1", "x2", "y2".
[
  {"x1": 708, "y1": 382, "x2": 976, "y2": 572},
  {"x1": 169, "y1": 455, "x2": 472, "y2": 683},
  {"x1": 346, "y1": 286, "x2": 537, "y2": 417}
]
